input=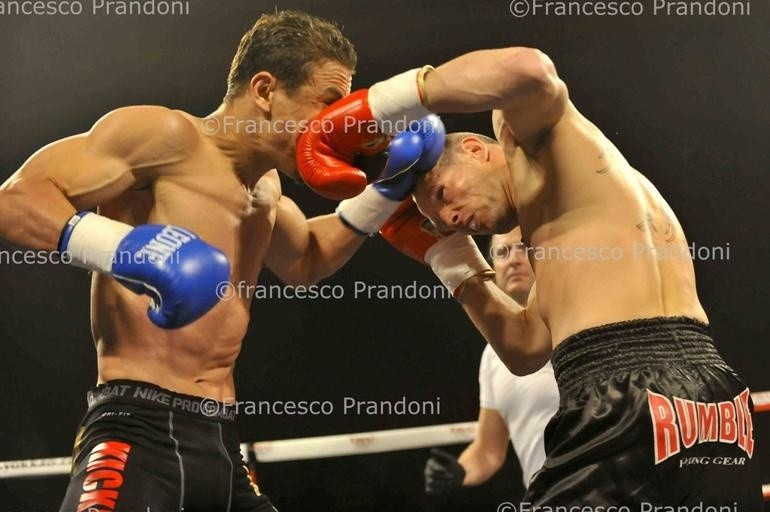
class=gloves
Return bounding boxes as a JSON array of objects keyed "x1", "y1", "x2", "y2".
[
  {"x1": 378, "y1": 195, "x2": 496, "y2": 303},
  {"x1": 294, "y1": 64, "x2": 433, "y2": 201},
  {"x1": 335, "y1": 113, "x2": 447, "y2": 237},
  {"x1": 56, "y1": 205, "x2": 229, "y2": 330},
  {"x1": 423, "y1": 447, "x2": 466, "y2": 497}
]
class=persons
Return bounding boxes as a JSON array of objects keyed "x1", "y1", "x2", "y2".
[
  {"x1": 422, "y1": 221, "x2": 562, "y2": 497},
  {"x1": 296, "y1": 46, "x2": 766, "y2": 512},
  {"x1": 1, "y1": 13, "x2": 445, "y2": 512}
]
